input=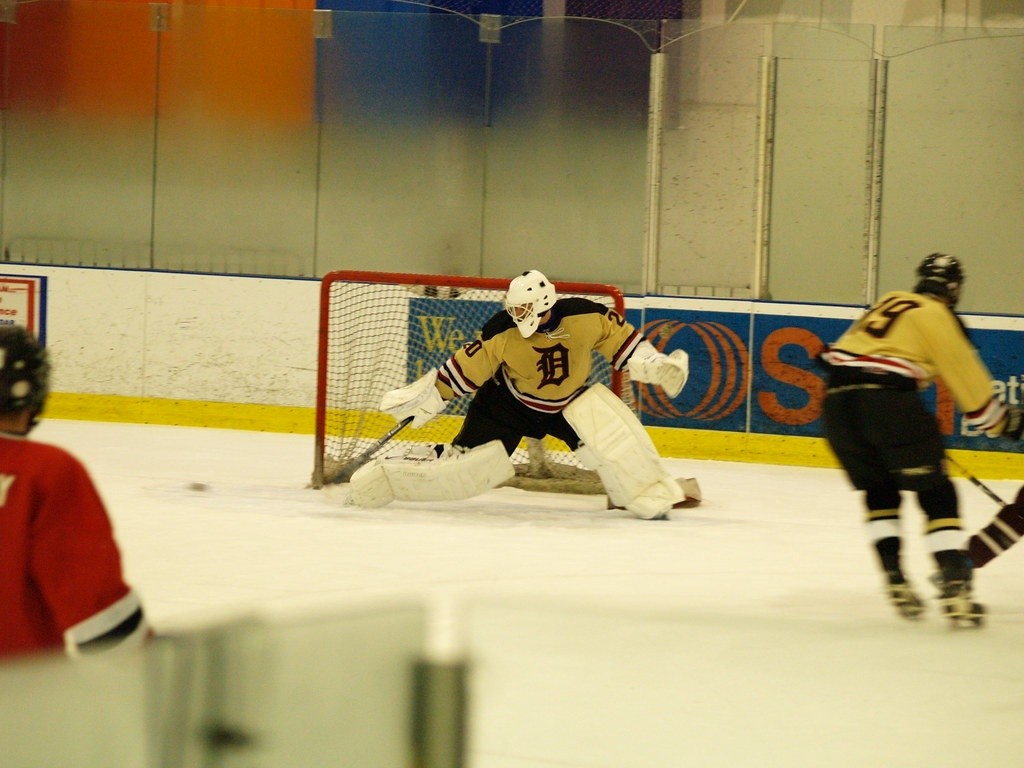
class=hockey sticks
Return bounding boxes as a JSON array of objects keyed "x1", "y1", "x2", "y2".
[
  {"x1": 320, "y1": 415, "x2": 415, "y2": 485},
  {"x1": 947, "y1": 454, "x2": 1007, "y2": 508}
]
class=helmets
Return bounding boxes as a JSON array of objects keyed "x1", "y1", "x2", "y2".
[
  {"x1": 0, "y1": 325, "x2": 49, "y2": 416},
  {"x1": 504, "y1": 269, "x2": 558, "y2": 338},
  {"x1": 915, "y1": 252, "x2": 965, "y2": 284}
]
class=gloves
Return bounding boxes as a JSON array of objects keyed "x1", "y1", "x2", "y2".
[{"x1": 1000, "y1": 408, "x2": 1024, "y2": 441}]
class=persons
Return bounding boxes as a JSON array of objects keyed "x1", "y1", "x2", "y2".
[
  {"x1": 349, "y1": 270, "x2": 689, "y2": 520},
  {"x1": 0, "y1": 324, "x2": 157, "y2": 671},
  {"x1": 815, "y1": 252, "x2": 1024, "y2": 631}
]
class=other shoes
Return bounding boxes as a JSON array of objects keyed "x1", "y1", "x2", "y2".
[
  {"x1": 884, "y1": 576, "x2": 927, "y2": 623},
  {"x1": 939, "y1": 548, "x2": 988, "y2": 632}
]
